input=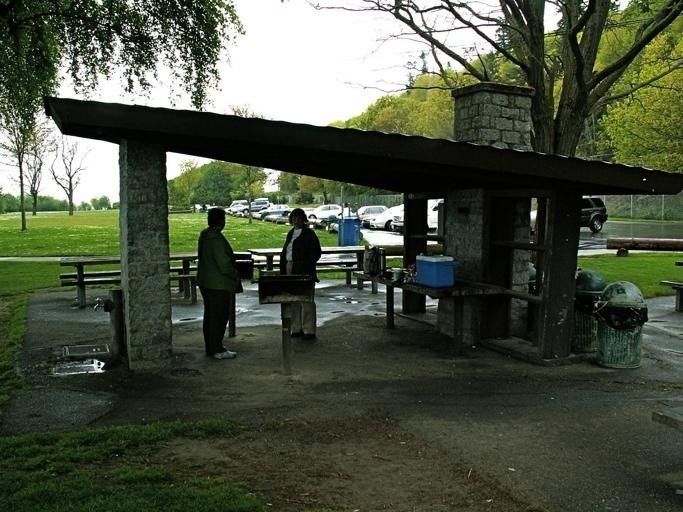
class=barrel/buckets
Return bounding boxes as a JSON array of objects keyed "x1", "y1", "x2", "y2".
[
  {"x1": 527, "y1": 281, "x2": 539, "y2": 341},
  {"x1": 363, "y1": 246, "x2": 387, "y2": 276},
  {"x1": 593, "y1": 299, "x2": 648, "y2": 368},
  {"x1": 573, "y1": 289, "x2": 603, "y2": 353}
]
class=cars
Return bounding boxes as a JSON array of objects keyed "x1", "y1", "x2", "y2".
[{"x1": 224, "y1": 197, "x2": 444, "y2": 230}]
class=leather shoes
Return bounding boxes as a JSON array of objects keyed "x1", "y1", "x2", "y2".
[
  {"x1": 301, "y1": 334, "x2": 315, "y2": 341},
  {"x1": 291, "y1": 332, "x2": 303, "y2": 337}
]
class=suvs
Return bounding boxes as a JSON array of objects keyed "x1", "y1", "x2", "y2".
[{"x1": 581, "y1": 197, "x2": 608, "y2": 232}]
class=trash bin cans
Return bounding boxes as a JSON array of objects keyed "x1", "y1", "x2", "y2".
[{"x1": 526, "y1": 262, "x2": 648, "y2": 370}]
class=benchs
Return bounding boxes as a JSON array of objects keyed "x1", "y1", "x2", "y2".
[
  {"x1": 58, "y1": 266, "x2": 198, "y2": 308},
  {"x1": 253, "y1": 260, "x2": 379, "y2": 294}
]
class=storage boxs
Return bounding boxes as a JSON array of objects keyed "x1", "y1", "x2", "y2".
[{"x1": 416, "y1": 255, "x2": 455, "y2": 289}]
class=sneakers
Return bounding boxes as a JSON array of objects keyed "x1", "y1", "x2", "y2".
[{"x1": 214, "y1": 350, "x2": 236, "y2": 359}]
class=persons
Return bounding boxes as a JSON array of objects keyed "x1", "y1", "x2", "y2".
[
  {"x1": 195, "y1": 206, "x2": 244, "y2": 361},
  {"x1": 279, "y1": 207, "x2": 322, "y2": 343}
]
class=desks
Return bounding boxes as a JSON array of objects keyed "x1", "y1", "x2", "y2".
[
  {"x1": 59, "y1": 254, "x2": 198, "y2": 299},
  {"x1": 248, "y1": 247, "x2": 365, "y2": 290}
]
